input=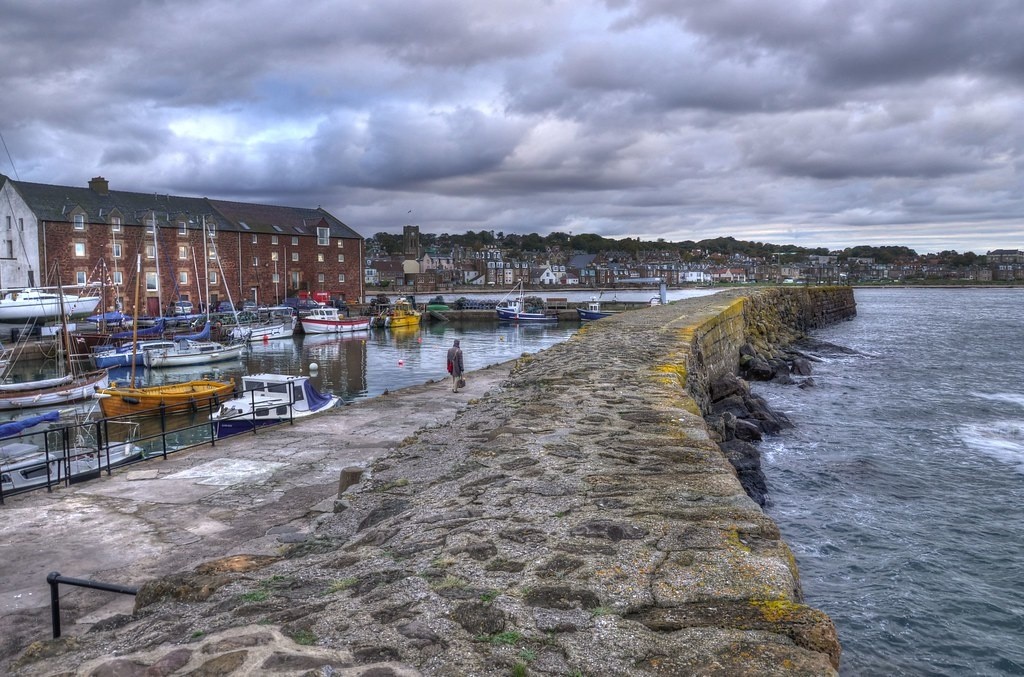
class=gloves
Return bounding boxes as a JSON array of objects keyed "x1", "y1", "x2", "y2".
[{"x1": 461, "y1": 369, "x2": 464, "y2": 372}]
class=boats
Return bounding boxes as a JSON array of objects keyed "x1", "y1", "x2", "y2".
[
  {"x1": 370, "y1": 290, "x2": 423, "y2": 328},
  {"x1": 575, "y1": 296, "x2": 612, "y2": 321},
  {"x1": 495, "y1": 277, "x2": 558, "y2": 321},
  {"x1": 300, "y1": 301, "x2": 375, "y2": 334},
  {"x1": 208, "y1": 372, "x2": 346, "y2": 440},
  {"x1": 0, "y1": 407, "x2": 144, "y2": 498}
]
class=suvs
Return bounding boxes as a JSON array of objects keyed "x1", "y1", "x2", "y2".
[
  {"x1": 165, "y1": 300, "x2": 194, "y2": 317},
  {"x1": 212, "y1": 300, "x2": 232, "y2": 312}
]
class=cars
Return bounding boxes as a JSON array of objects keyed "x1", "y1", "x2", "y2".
[
  {"x1": 297, "y1": 299, "x2": 319, "y2": 309},
  {"x1": 236, "y1": 300, "x2": 257, "y2": 312}
]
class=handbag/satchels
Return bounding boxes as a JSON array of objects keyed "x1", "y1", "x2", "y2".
[
  {"x1": 447, "y1": 360, "x2": 453, "y2": 373},
  {"x1": 457, "y1": 371, "x2": 465, "y2": 388}
]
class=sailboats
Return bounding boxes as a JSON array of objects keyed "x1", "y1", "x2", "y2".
[{"x1": 0, "y1": 212, "x2": 299, "y2": 423}]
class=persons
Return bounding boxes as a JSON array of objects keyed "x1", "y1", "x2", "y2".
[
  {"x1": 198, "y1": 300, "x2": 206, "y2": 314},
  {"x1": 447, "y1": 340, "x2": 464, "y2": 392}
]
